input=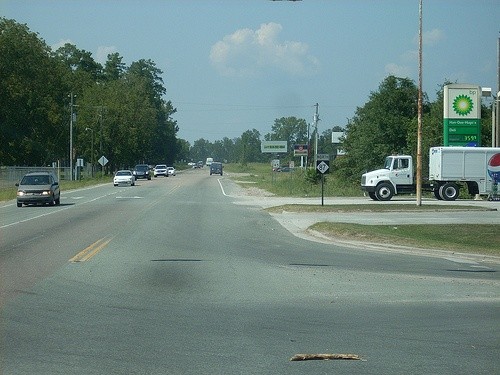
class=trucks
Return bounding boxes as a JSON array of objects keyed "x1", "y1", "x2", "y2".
[{"x1": 205, "y1": 157, "x2": 214, "y2": 166}]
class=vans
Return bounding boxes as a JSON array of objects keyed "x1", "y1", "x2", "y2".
[{"x1": 210, "y1": 161, "x2": 225, "y2": 176}]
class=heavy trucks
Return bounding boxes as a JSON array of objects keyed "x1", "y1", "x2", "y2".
[{"x1": 361, "y1": 146, "x2": 499, "y2": 201}]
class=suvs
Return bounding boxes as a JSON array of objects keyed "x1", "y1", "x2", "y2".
[{"x1": 133, "y1": 163, "x2": 152, "y2": 181}]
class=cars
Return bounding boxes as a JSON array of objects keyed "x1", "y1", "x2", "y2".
[
  {"x1": 113, "y1": 170, "x2": 135, "y2": 186},
  {"x1": 167, "y1": 166, "x2": 176, "y2": 177},
  {"x1": 278, "y1": 167, "x2": 291, "y2": 173},
  {"x1": 153, "y1": 164, "x2": 167, "y2": 177},
  {"x1": 14, "y1": 172, "x2": 60, "y2": 207}
]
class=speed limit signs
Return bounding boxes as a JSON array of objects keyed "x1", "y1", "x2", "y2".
[{"x1": 316, "y1": 161, "x2": 330, "y2": 175}]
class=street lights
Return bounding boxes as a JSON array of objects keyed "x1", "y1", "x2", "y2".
[{"x1": 84, "y1": 126, "x2": 94, "y2": 178}]
